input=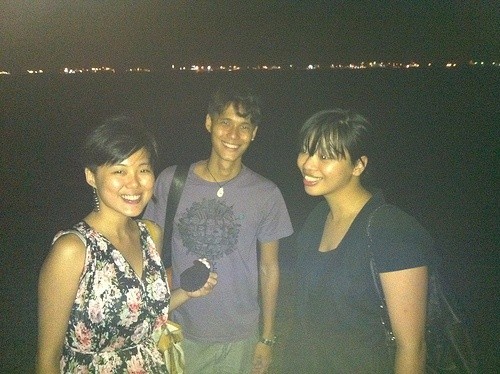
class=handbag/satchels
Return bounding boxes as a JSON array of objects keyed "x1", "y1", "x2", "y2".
[
  {"x1": 366, "y1": 203, "x2": 480, "y2": 374},
  {"x1": 157, "y1": 320, "x2": 186, "y2": 374}
]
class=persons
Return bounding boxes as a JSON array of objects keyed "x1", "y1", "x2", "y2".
[
  {"x1": 35, "y1": 117, "x2": 217, "y2": 374},
  {"x1": 142, "y1": 83, "x2": 294, "y2": 374},
  {"x1": 282, "y1": 109, "x2": 428, "y2": 374}
]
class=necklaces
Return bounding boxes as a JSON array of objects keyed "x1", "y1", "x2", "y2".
[{"x1": 206, "y1": 161, "x2": 242, "y2": 198}]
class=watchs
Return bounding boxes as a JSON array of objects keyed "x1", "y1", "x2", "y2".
[{"x1": 260, "y1": 336, "x2": 277, "y2": 348}]
